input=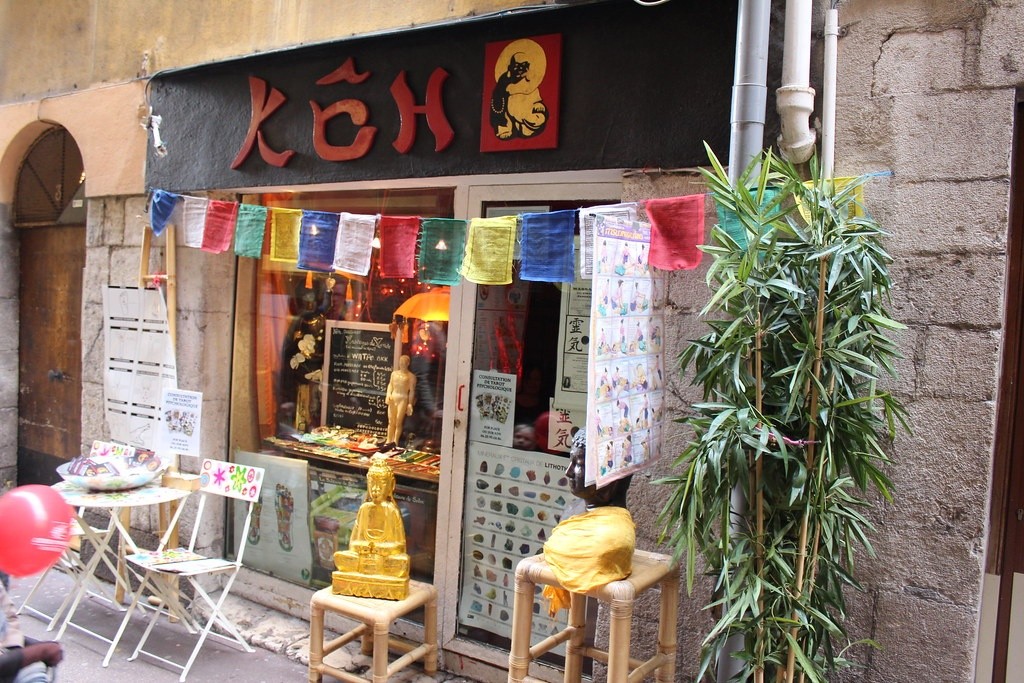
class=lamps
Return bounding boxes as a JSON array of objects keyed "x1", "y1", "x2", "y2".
[{"x1": 389, "y1": 291, "x2": 450, "y2": 348}]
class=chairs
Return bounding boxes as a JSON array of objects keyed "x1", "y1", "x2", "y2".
[
  {"x1": 123, "y1": 457, "x2": 265, "y2": 683},
  {"x1": 16, "y1": 507, "x2": 122, "y2": 632}
]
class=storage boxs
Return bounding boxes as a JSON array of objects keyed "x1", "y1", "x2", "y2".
[{"x1": 161, "y1": 472, "x2": 201, "y2": 492}]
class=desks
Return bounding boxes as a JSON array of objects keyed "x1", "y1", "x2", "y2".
[{"x1": 51, "y1": 476, "x2": 194, "y2": 666}]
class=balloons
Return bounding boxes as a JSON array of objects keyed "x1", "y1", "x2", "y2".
[{"x1": 0, "y1": 484, "x2": 71, "y2": 579}]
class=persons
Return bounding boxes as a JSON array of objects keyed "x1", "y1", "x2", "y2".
[
  {"x1": 0, "y1": 580, "x2": 48, "y2": 683},
  {"x1": 543, "y1": 424, "x2": 635, "y2": 574},
  {"x1": 0, "y1": 641, "x2": 63, "y2": 683},
  {"x1": 511, "y1": 424, "x2": 540, "y2": 453},
  {"x1": 384, "y1": 355, "x2": 417, "y2": 447},
  {"x1": 286, "y1": 292, "x2": 327, "y2": 383},
  {"x1": 333, "y1": 458, "x2": 411, "y2": 577}
]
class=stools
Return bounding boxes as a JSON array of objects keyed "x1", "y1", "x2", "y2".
[
  {"x1": 507, "y1": 551, "x2": 678, "y2": 683},
  {"x1": 308, "y1": 578, "x2": 439, "y2": 683}
]
